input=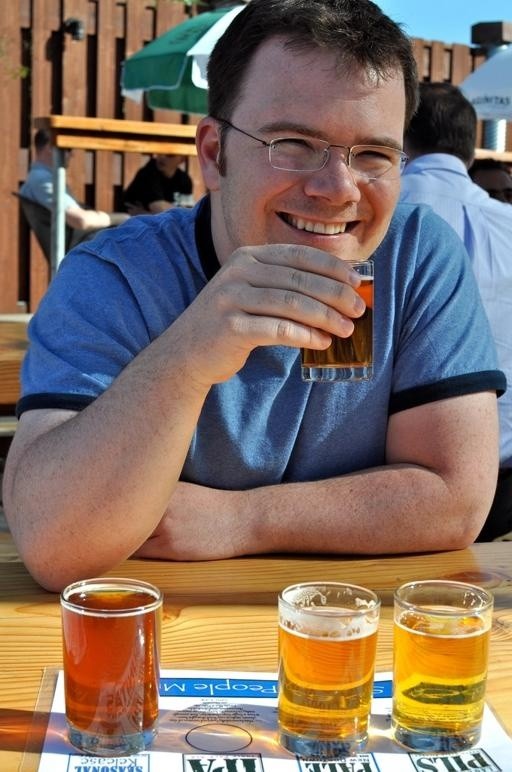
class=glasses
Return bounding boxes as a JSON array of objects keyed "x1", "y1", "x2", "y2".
[{"x1": 210, "y1": 112, "x2": 410, "y2": 183}]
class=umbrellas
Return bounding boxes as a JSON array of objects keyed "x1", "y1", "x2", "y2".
[
  {"x1": 464, "y1": 45, "x2": 512, "y2": 124},
  {"x1": 121, "y1": 4, "x2": 251, "y2": 115}
]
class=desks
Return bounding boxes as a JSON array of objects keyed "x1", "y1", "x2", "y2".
[{"x1": 0, "y1": 540, "x2": 512, "y2": 772}]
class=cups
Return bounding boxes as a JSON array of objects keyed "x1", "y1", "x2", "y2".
[
  {"x1": 59, "y1": 574, "x2": 164, "y2": 757},
  {"x1": 391, "y1": 573, "x2": 493, "y2": 751},
  {"x1": 294, "y1": 248, "x2": 375, "y2": 383},
  {"x1": 273, "y1": 578, "x2": 377, "y2": 755}
]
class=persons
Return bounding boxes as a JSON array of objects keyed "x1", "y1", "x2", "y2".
[
  {"x1": 473, "y1": 158, "x2": 511, "y2": 206},
  {"x1": 399, "y1": 78, "x2": 509, "y2": 540},
  {"x1": 19, "y1": 127, "x2": 137, "y2": 251},
  {"x1": 2, "y1": 0, "x2": 508, "y2": 594},
  {"x1": 117, "y1": 154, "x2": 195, "y2": 217}
]
